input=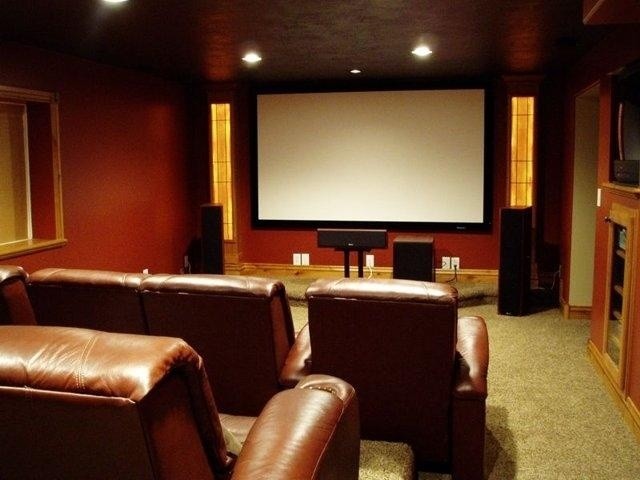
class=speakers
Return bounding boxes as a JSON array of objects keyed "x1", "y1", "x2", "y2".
[
  {"x1": 497, "y1": 206, "x2": 533, "y2": 315},
  {"x1": 316, "y1": 227, "x2": 389, "y2": 250},
  {"x1": 200, "y1": 202, "x2": 225, "y2": 275},
  {"x1": 392, "y1": 235, "x2": 436, "y2": 280}
]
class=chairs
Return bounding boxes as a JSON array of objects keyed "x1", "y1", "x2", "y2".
[
  {"x1": 0, "y1": 263, "x2": 36, "y2": 326},
  {"x1": 278, "y1": 274, "x2": 489, "y2": 480}
]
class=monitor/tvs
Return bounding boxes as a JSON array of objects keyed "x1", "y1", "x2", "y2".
[
  {"x1": 612, "y1": 81, "x2": 640, "y2": 185},
  {"x1": 250, "y1": 81, "x2": 494, "y2": 236}
]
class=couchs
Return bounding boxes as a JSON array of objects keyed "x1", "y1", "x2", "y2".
[
  {"x1": 22, "y1": 267, "x2": 297, "y2": 416},
  {"x1": 0, "y1": 323, "x2": 362, "y2": 480}
]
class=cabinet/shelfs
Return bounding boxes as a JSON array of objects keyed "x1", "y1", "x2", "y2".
[{"x1": 586, "y1": 177, "x2": 640, "y2": 443}]
